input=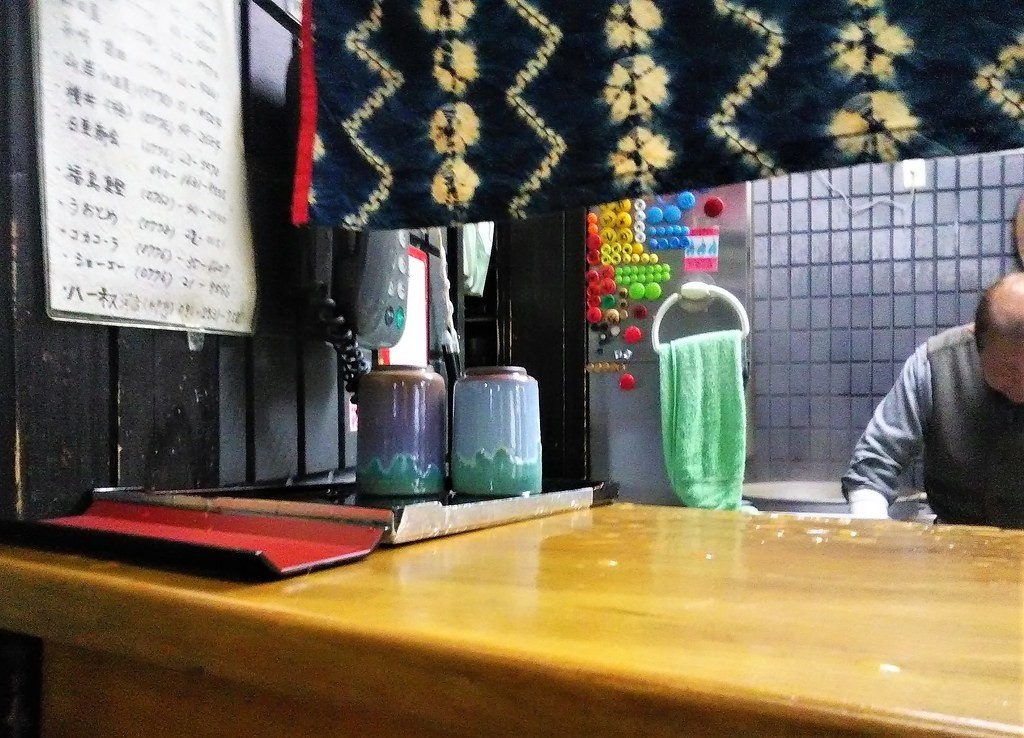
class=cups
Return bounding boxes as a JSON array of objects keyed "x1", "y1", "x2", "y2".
[
  {"x1": 452, "y1": 366, "x2": 541, "y2": 494},
  {"x1": 358, "y1": 364, "x2": 446, "y2": 495}
]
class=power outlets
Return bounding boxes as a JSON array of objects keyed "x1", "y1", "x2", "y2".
[{"x1": 905, "y1": 158, "x2": 927, "y2": 188}]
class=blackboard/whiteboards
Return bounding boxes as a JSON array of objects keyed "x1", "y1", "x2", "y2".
[{"x1": 30, "y1": 0, "x2": 261, "y2": 338}]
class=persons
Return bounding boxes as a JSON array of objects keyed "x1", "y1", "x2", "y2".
[{"x1": 840, "y1": 274, "x2": 1024, "y2": 528}]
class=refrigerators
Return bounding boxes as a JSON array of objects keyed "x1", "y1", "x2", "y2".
[{"x1": 587, "y1": 181, "x2": 753, "y2": 510}]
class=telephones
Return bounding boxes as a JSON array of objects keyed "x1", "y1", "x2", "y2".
[{"x1": 293, "y1": 229, "x2": 409, "y2": 348}]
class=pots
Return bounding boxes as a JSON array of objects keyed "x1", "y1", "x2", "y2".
[{"x1": 740, "y1": 472, "x2": 928, "y2": 522}]
